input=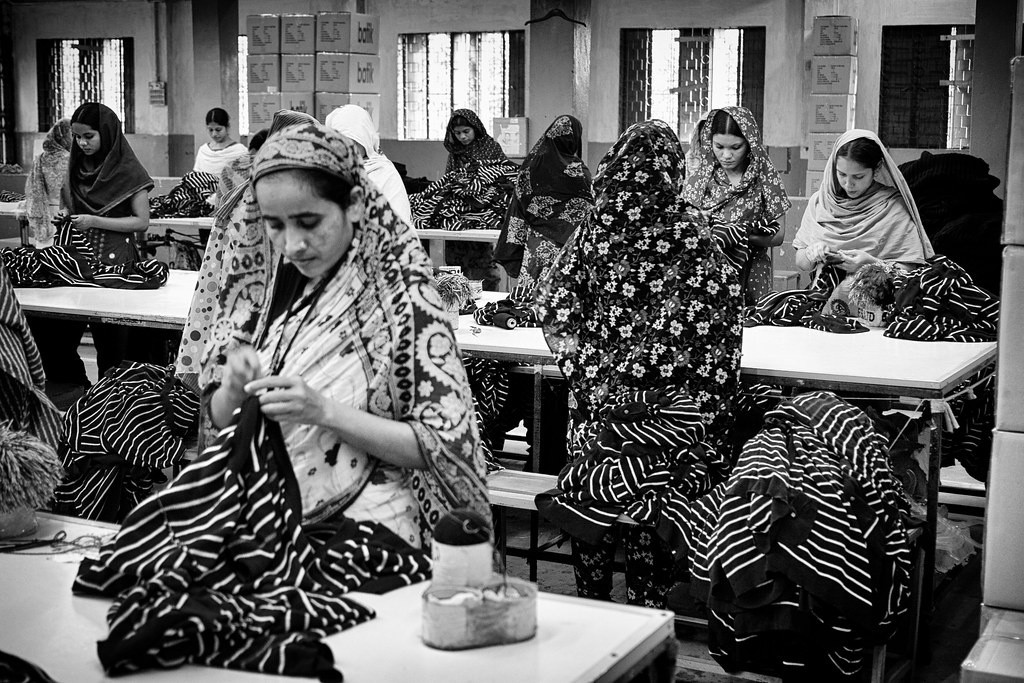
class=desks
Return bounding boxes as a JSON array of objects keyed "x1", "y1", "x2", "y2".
[{"x1": 0, "y1": 200, "x2": 1000, "y2": 683}]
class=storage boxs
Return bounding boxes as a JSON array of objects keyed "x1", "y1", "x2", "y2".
[
  {"x1": 247, "y1": 10, "x2": 380, "y2": 146},
  {"x1": 493, "y1": 117, "x2": 528, "y2": 159},
  {"x1": 805, "y1": 14, "x2": 859, "y2": 200}
]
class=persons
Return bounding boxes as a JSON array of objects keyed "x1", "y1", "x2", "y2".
[
  {"x1": 683, "y1": 115, "x2": 708, "y2": 180},
  {"x1": 193, "y1": 106, "x2": 249, "y2": 247},
  {"x1": 493, "y1": 113, "x2": 595, "y2": 302},
  {"x1": 534, "y1": 114, "x2": 749, "y2": 613},
  {"x1": 213, "y1": 107, "x2": 325, "y2": 213},
  {"x1": 325, "y1": 103, "x2": 412, "y2": 226},
  {"x1": 442, "y1": 107, "x2": 506, "y2": 285},
  {"x1": 197, "y1": 122, "x2": 473, "y2": 560},
  {"x1": 23, "y1": 118, "x2": 73, "y2": 253},
  {"x1": 679, "y1": 104, "x2": 793, "y2": 306},
  {"x1": 51, "y1": 100, "x2": 155, "y2": 389},
  {"x1": 791, "y1": 128, "x2": 934, "y2": 284}
]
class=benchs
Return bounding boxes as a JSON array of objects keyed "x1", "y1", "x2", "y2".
[
  {"x1": 482, "y1": 468, "x2": 929, "y2": 683},
  {"x1": 773, "y1": 272, "x2": 802, "y2": 293}
]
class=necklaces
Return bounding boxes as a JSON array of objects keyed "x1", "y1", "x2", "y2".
[{"x1": 268, "y1": 244, "x2": 353, "y2": 392}]
